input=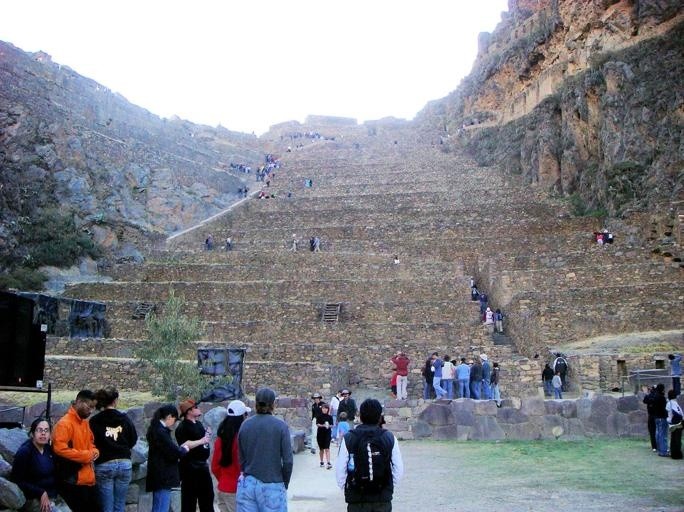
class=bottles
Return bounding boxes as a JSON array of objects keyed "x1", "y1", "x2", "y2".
[
  {"x1": 203, "y1": 427, "x2": 214, "y2": 448},
  {"x1": 348, "y1": 454, "x2": 356, "y2": 486}
]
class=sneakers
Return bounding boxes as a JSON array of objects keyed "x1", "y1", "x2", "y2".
[
  {"x1": 319, "y1": 461, "x2": 325, "y2": 467},
  {"x1": 325, "y1": 462, "x2": 333, "y2": 469},
  {"x1": 652, "y1": 448, "x2": 671, "y2": 458}
]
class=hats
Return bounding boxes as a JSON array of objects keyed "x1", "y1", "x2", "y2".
[
  {"x1": 179, "y1": 399, "x2": 196, "y2": 418},
  {"x1": 479, "y1": 353, "x2": 488, "y2": 361},
  {"x1": 310, "y1": 392, "x2": 322, "y2": 399},
  {"x1": 226, "y1": 399, "x2": 252, "y2": 417},
  {"x1": 340, "y1": 389, "x2": 352, "y2": 397}
]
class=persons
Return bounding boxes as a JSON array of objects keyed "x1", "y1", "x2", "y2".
[
  {"x1": 553, "y1": 354, "x2": 568, "y2": 391},
  {"x1": 390, "y1": 352, "x2": 502, "y2": 408},
  {"x1": 225, "y1": 236, "x2": 232, "y2": 251},
  {"x1": 312, "y1": 236, "x2": 320, "y2": 252},
  {"x1": 596, "y1": 232, "x2": 603, "y2": 244},
  {"x1": 289, "y1": 234, "x2": 298, "y2": 252},
  {"x1": 469, "y1": 276, "x2": 503, "y2": 333},
  {"x1": 604, "y1": 230, "x2": 613, "y2": 244},
  {"x1": 552, "y1": 372, "x2": 563, "y2": 399},
  {"x1": 309, "y1": 236, "x2": 314, "y2": 251},
  {"x1": 642, "y1": 354, "x2": 684, "y2": 459},
  {"x1": 336, "y1": 399, "x2": 404, "y2": 512},
  {"x1": 207, "y1": 235, "x2": 213, "y2": 250},
  {"x1": 542, "y1": 364, "x2": 554, "y2": 396},
  {"x1": 229, "y1": 131, "x2": 334, "y2": 200}
]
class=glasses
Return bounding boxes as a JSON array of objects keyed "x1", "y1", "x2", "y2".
[{"x1": 35, "y1": 428, "x2": 50, "y2": 432}]
